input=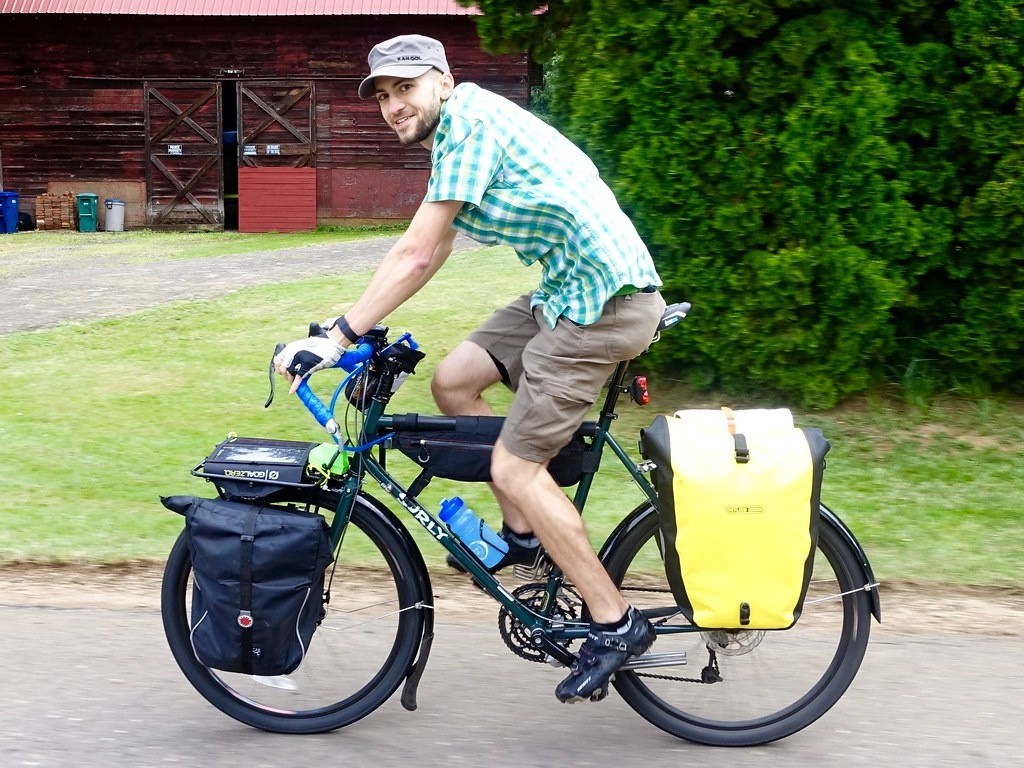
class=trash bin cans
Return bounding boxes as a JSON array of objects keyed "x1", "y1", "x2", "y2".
[
  {"x1": 0, "y1": 190, "x2": 22, "y2": 236},
  {"x1": 75, "y1": 190, "x2": 127, "y2": 234}
]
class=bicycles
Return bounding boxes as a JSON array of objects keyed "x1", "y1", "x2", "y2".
[{"x1": 159, "y1": 300, "x2": 881, "y2": 750}]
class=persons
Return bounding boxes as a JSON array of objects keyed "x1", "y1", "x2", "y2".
[{"x1": 272, "y1": 34, "x2": 667, "y2": 705}]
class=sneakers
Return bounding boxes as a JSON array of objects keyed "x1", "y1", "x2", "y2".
[
  {"x1": 555, "y1": 606, "x2": 657, "y2": 704},
  {"x1": 447, "y1": 528, "x2": 544, "y2": 575}
]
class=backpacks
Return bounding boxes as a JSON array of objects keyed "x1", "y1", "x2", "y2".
[
  {"x1": 640, "y1": 406, "x2": 832, "y2": 631},
  {"x1": 160, "y1": 496, "x2": 334, "y2": 676}
]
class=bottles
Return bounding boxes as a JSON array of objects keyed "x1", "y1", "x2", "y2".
[{"x1": 441, "y1": 498, "x2": 510, "y2": 570}]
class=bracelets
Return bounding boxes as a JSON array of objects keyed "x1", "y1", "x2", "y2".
[{"x1": 335, "y1": 316, "x2": 364, "y2": 345}]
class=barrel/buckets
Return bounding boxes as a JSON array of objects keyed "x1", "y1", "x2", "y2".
[
  {"x1": 0, "y1": 193, "x2": 19, "y2": 234},
  {"x1": 105, "y1": 199, "x2": 126, "y2": 232}
]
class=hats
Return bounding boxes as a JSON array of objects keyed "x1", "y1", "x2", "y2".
[{"x1": 357, "y1": 33, "x2": 449, "y2": 99}]
